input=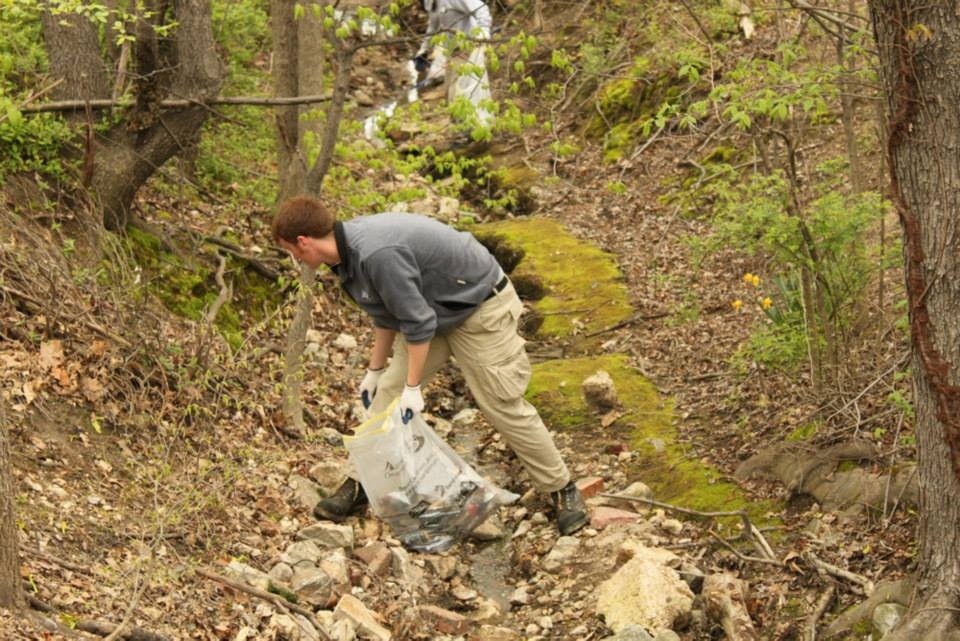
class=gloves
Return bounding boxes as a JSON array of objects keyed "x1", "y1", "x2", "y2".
[
  {"x1": 399, "y1": 385, "x2": 424, "y2": 423},
  {"x1": 415, "y1": 48, "x2": 428, "y2": 72},
  {"x1": 360, "y1": 368, "x2": 385, "y2": 409}
]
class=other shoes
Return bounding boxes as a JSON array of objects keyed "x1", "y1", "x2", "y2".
[
  {"x1": 464, "y1": 143, "x2": 491, "y2": 157},
  {"x1": 451, "y1": 137, "x2": 471, "y2": 145}
]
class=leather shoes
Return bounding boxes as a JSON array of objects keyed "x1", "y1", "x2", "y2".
[
  {"x1": 315, "y1": 478, "x2": 367, "y2": 523},
  {"x1": 551, "y1": 486, "x2": 591, "y2": 534}
]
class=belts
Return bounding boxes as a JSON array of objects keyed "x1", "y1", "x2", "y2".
[{"x1": 485, "y1": 277, "x2": 508, "y2": 302}]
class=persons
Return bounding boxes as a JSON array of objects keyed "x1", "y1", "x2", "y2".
[
  {"x1": 271, "y1": 195, "x2": 588, "y2": 536},
  {"x1": 415, "y1": 0, "x2": 497, "y2": 147}
]
